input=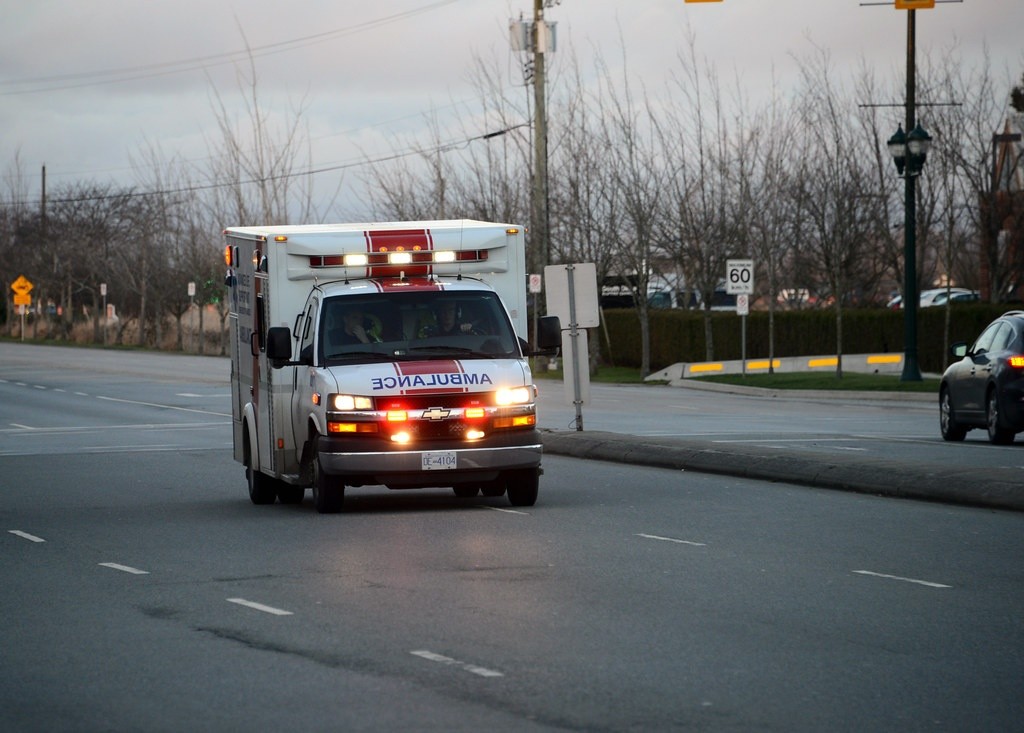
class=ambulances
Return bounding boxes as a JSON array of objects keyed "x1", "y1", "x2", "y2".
[{"x1": 225, "y1": 218, "x2": 565, "y2": 513}]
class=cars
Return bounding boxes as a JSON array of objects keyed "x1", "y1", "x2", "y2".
[{"x1": 887, "y1": 282, "x2": 979, "y2": 305}]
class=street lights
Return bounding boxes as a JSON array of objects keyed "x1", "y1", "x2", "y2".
[{"x1": 885, "y1": 120, "x2": 933, "y2": 380}]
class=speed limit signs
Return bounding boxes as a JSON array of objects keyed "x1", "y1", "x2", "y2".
[{"x1": 725, "y1": 259, "x2": 753, "y2": 294}]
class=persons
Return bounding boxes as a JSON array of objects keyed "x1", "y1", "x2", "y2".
[
  {"x1": 321, "y1": 307, "x2": 382, "y2": 345},
  {"x1": 420, "y1": 295, "x2": 472, "y2": 341}
]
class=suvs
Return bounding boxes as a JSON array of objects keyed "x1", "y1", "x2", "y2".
[{"x1": 939, "y1": 311, "x2": 1024, "y2": 443}]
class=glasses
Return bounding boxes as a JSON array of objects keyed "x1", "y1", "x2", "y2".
[{"x1": 436, "y1": 308, "x2": 457, "y2": 313}]
